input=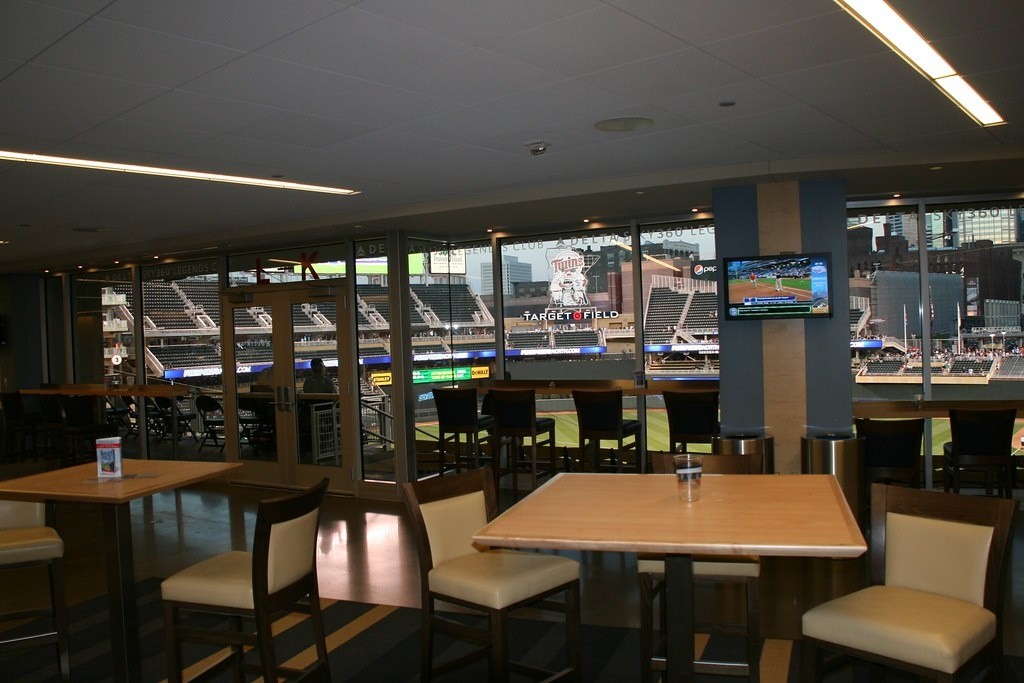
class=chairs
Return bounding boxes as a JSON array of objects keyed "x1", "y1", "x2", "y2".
[
  {"x1": 661, "y1": 390, "x2": 721, "y2": 454},
  {"x1": 1, "y1": 526, "x2": 69, "y2": 681},
  {"x1": 400, "y1": 464, "x2": 585, "y2": 682},
  {"x1": 854, "y1": 418, "x2": 927, "y2": 488},
  {"x1": 943, "y1": 409, "x2": 1018, "y2": 500},
  {"x1": 636, "y1": 553, "x2": 777, "y2": 682},
  {"x1": 801, "y1": 483, "x2": 1021, "y2": 682},
  {"x1": 2, "y1": 389, "x2": 104, "y2": 462},
  {"x1": 571, "y1": 389, "x2": 643, "y2": 475},
  {"x1": 105, "y1": 395, "x2": 275, "y2": 455},
  {"x1": 432, "y1": 387, "x2": 494, "y2": 477},
  {"x1": 160, "y1": 475, "x2": 333, "y2": 681},
  {"x1": 487, "y1": 389, "x2": 560, "y2": 489}
]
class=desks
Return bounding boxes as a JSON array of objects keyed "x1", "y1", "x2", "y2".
[
  {"x1": 491, "y1": 379, "x2": 721, "y2": 398},
  {"x1": 471, "y1": 472, "x2": 869, "y2": 682},
  {"x1": 852, "y1": 399, "x2": 1023, "y2": 420},
  {"x1": 2, "y1": 457, "x2": 245, "y2": 681}
]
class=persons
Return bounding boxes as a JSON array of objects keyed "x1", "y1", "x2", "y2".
[
  {"x1": 749, "y1": 272, "x2": 756, "y2": 287},
  {"x1": 250, "y1": 363, "x2": 275, "y2": 432},
  {"x1": 773, "y1": 267, "x2": 782, "y2": 291},
  {"x1": 851, "y1": 330, "x2": 1023, "y2": 377},
  {"x1": 645, "y1": 309, "x2": 720, "y2": 370},
  {"x1": 303, "y1": 358, "x2": 340, "y2": 447},
  {"x1": 244, "y1": 328, "x2": 496, "y2": 346},
  {"x1": 504, "y1": 323, "x2": 635, "y2": 364}
]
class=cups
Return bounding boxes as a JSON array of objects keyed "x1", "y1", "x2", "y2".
[
  {"x1": 675, "y1": 454, "x2": 703, "y2": 501},
  {"x1": 127, "y1": 377, "x2": 134, "y2": 391},
  {"x1": 104, "y1": 376, "x2": 112, "y2": 392}
]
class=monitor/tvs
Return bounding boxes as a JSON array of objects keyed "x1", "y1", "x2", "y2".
[{"x1": 723, "y1": 252, "x2": 834, "y2": 321}]
